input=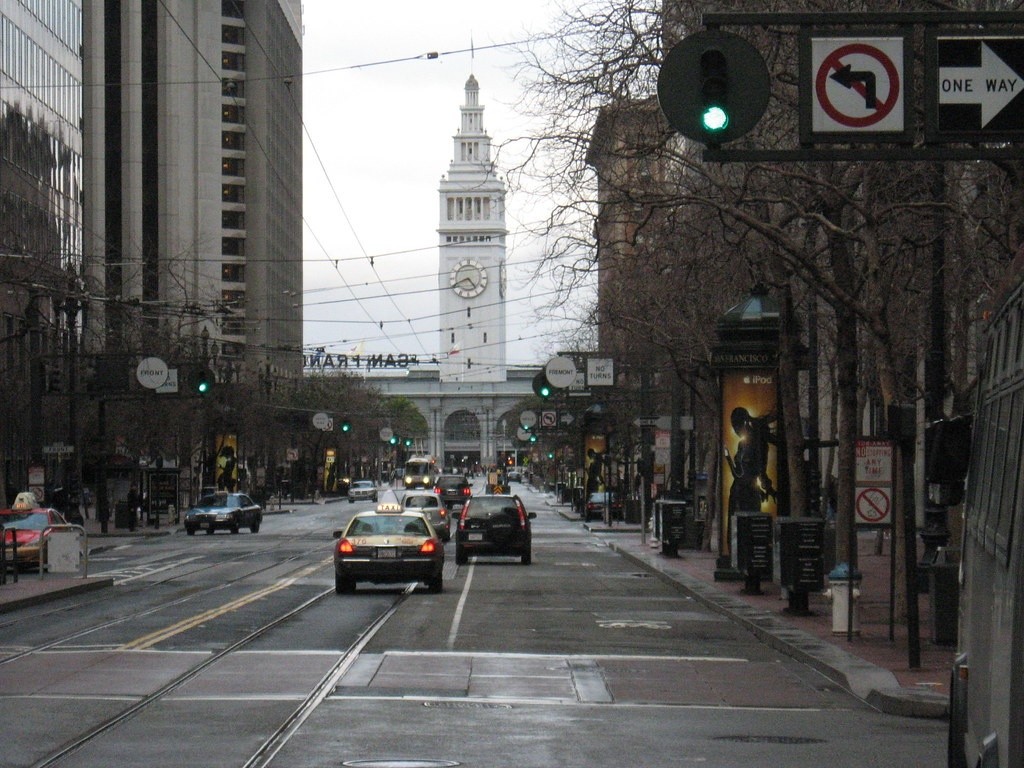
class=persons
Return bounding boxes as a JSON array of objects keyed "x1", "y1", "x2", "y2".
[
  {"x1": 167, "y1": 489, "x2": 176, "y2": 527},
  {"x1": 127, "y1": 483, "x2": 139, "y2": 532}
]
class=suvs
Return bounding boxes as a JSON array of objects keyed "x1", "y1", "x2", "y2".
[
  {"x1": 434, "y1": 476, "x2": 473, "y2": 507},
  {"x1": 452, "y1": 494, "x2": 537, "y2": 565}
]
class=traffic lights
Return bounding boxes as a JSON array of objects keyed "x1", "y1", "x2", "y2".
[
  {"x1": 702, "y1": 49, "x2": 730, "y2": 134},
  {"x1": 198, "y1": 370, "x2": 207, "y2": 393},
  {"x1": 530, "y1": 434, "x2": 536, "y2": 442},
  {"x1": 342, "y1": 419, "x2": 411, "y2": 446},
  {"x1": 548, "y1": 453, "x2": 553, "y2": 458},
  {"x1": 524, "y1": 424, "x2": 528, "y2": 430},
  {"x1": 541, "y1": 375, "x2": 551, "y2": 397},
  {"x1": 48, "y1": 367, "x2": 60, "y2": 391}
]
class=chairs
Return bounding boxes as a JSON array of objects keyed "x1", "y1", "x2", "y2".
[
  {"x1": 361, "y1": 521, "x2": 380, "y2": 533},
  {"x1": 404, "y1": 522, "x2": 420, "y2": 533}
]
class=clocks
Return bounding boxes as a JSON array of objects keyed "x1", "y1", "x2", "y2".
[{"x1": 449, "y1": 259, "x2": 488, "y2": 299}]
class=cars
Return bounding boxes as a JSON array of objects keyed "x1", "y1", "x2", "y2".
[
  {"x1": 508, "y1": 471, "x2": 521, "y2": 483},
  {"x1": 184, "y1": 493, "x2": 262, "y2": 535},
  {"x1": 402, "y1": 492, "x2": 451, "y2": 541},
  {"x1": 0, "y1": 508, "x2": 67, "y2": 570},
  {"x1": 332, "y1": 508, "x2": 445, "y2": 595},
  {"x1": 348, "y1": 481, "x2": 378, "y2": 502},
  {"x1": 584, "y1": 492, "x2": 623, "y2": 522}
]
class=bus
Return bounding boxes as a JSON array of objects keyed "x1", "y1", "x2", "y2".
[
  {"x1": 403, "y1": 455, "x2": 437, "y2": 489},
  {"x1": 923, "y1": 240, "x2": 1024, "y2": 768}
]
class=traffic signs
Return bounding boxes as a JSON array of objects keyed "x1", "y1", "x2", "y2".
[{"x1": 937, "y1": 35, "x2": 1024, "y2": 132}]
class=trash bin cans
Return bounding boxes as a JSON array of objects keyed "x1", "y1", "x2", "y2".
[
  {"x1": 932, "y1": 546, "x2": 962, "y2": 649},
  {"x1": 115, "y1": 500, "x2": 132, "y2": 529}
]
